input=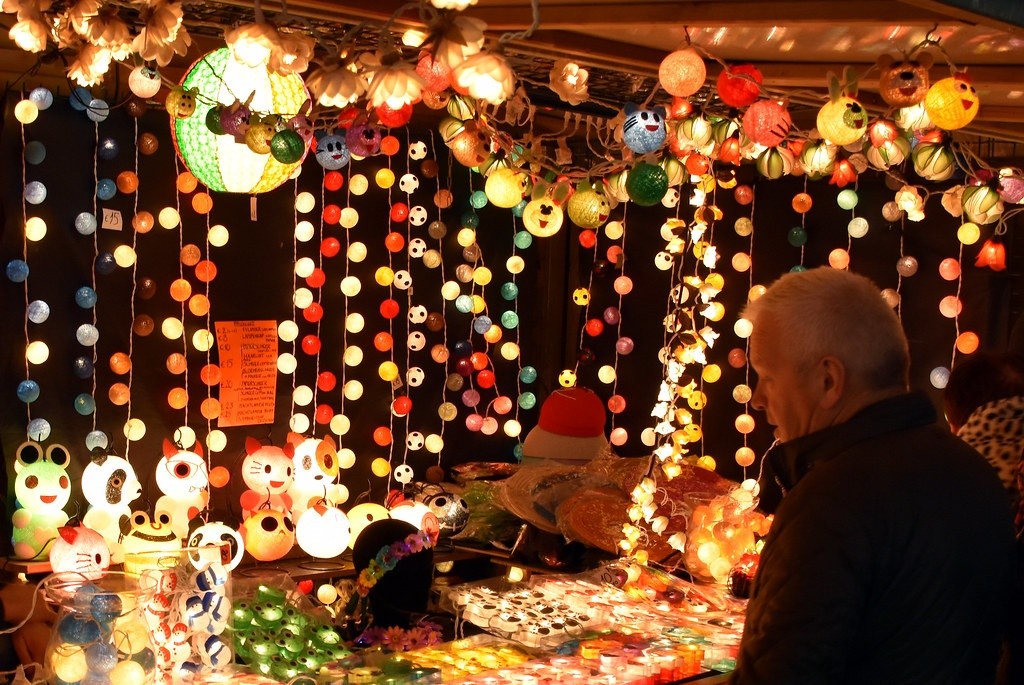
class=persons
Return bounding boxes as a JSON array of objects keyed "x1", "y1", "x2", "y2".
[{"x1": 725, "y1": 264, "x2": 1024, "y2": 685}]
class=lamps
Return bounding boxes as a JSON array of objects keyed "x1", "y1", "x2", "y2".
[{"x1": 2, "y1": 4, "x2": 1023, "y2": 685}]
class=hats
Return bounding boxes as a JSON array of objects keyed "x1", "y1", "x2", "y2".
[{"x1": 518, "y1": 383, "x2": 614, "y2": 465}]
